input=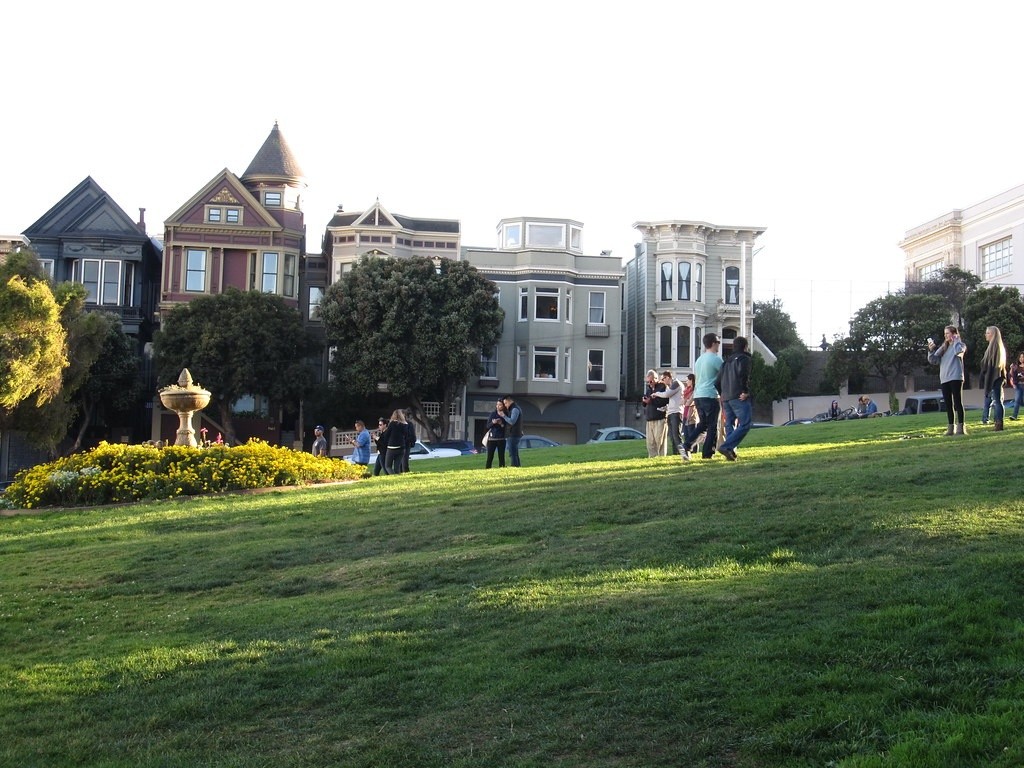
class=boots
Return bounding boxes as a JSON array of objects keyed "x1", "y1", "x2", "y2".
[
  {"x1": 955, "y1": 423, "x2": 964, "y2": 435},
  {"x1": 944, "y1": 424, "x2": 954, "y2": 436},
  {"x1": 993, "y1": 419, "x2": 1003, "y2": 431}
]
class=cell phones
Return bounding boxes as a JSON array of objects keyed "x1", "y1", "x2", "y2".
[
  {"x1": 927, "y1": 338, "x2": 934, "y2": 344},
  {"x1": 346, "y1": 434, "x2": 349, "y2": 439}
]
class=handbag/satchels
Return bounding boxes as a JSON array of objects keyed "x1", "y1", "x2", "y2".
[{"x1": 481, "y1": 431, "x2": 490, "y2": 447}]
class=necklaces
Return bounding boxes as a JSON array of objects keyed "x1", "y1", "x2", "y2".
[{"x1": 833, "y1": 409, "x2": 836, "y2": 415}]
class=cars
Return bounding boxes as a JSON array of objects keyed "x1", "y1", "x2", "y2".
[
  {"x1": 753, "y1": 423, "x2": 776, "y2": 428},
  {"x1": 586, "y1": 427, "x2": 646, "y2": 444},
  {"x1": 480, "y1": 435, "x2": 562, "y2": 453},
  {"x1": 780, "y1": 397, "x2": 1016, "y2": 426}
]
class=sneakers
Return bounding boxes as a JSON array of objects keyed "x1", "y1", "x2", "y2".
[
  {"x1": 717, "y1": 447, "x2": 737, "y2": 461},
  {"x1": 677, "y1": 444, "x2": 692, "y2": 461}
]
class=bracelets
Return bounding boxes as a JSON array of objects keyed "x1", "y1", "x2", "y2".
[{"x1": 352, "y1": 440, "x2": 355, "y2": 444}]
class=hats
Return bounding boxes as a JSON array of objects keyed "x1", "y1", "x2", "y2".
[{"x1": 313, "y1": 425, "x2": 324, "y2": 431}]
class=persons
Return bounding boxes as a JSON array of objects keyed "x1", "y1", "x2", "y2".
[
  {"x1": 311, "y1": 425, "x2": 327, "y2": 458},
  {"x1": 828, "y1": 395, "x2": 877, "y2": 419},
  {"x1": 981, "y1": 326, "x2": 1024, "y2": 431},
  {"x1": 485, "y1": 397, "x2": 508, "y2": 469},
  {"x1": 498, "y1": 395, "x2": 523, "y2": 467},
  {"x1": 348, "y1": 409, "x2": 416, "y2": 477},
  {"x1": 643, "y1": 332, "x2": 753, "y2": 461},
  {"x1": 928, "y1": 326, "x2": 968, "y2": 435}
]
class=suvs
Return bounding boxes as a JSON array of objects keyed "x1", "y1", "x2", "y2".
[
  {"x1": 424, "y1": 440, "x2": 479, "y2": 456},
  {"x1": 343, "y1": 440, "x2": 462, "y2": 466}
]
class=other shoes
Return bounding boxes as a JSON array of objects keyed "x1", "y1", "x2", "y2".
[{"x1": 1009, "y1": 416, "x2": 1017, "y2": 420}]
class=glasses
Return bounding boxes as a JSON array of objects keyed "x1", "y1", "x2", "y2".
[
  {"x1": 647, "y1": 381, "x2": 653, "y2": 383},
  {"x1": 378, "y1": 424, "x2": 386, "y2": 426},
  {"x1": 662, "y1": 377, "x2": 666, "y2": 381},
  {"x1": 498, "y1": 398, "x2": 503, "y2": 401}
]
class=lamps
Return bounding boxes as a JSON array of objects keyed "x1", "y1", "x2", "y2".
[{"x1": 636, "y1": 410, "x2": 641, "y2": 420}]
